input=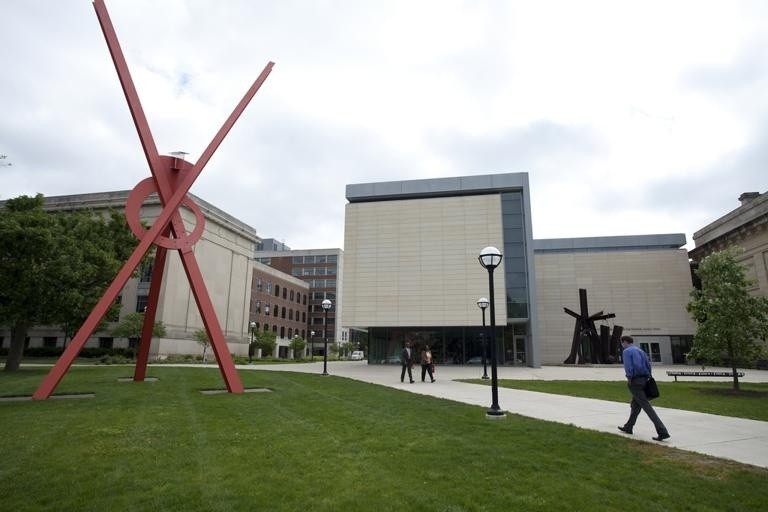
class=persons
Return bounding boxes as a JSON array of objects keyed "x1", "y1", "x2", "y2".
[
  {"x1": 617, "y1": 335, "x2": 670, "y2": 441},
  {"x1": 419, "y1": 345, "x2": 435, "y2": 383},
  {"x1": 400, "y1": 342, "x2": 415, "y2": 383}
]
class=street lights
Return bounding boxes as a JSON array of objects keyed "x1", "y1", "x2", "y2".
[
  {"x1": 478, "y1": 246, "x2": 507, "y2": 418},
  {"x1": 478, "y1": 298, "x2": 490, "y2": 379},
  {"x1": 310, "y1": 331, "x2": 316, "y2": 360},
  {"x1": 250, "y1": 321, "x2": 257, "y2": 363},
  {"x1": 295, "y1": 335, "x2": 299, "y2": 358},
  {"x1": 321, "y1": 300, "x2": 332, "y2": 375}
]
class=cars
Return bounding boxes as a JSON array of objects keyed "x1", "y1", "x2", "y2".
[
  {"x1": 467, "y1": 357, "x2": 489, "y2": 365},
  {"x1": 351, "y1": 351, "x2": 364, "y2": 360}
]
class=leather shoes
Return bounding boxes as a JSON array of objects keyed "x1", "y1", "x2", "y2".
[
  {"x1": 618, "y1": 427, "x2": 633, "y2": 434},
  {"x1": 653, "y1": 435, "x2": 670, "y2": 440}
]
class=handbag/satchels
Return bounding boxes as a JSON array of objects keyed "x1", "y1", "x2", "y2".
[{"x1": 643, "y1": 378, "x2": 659, "y2": 400}]
